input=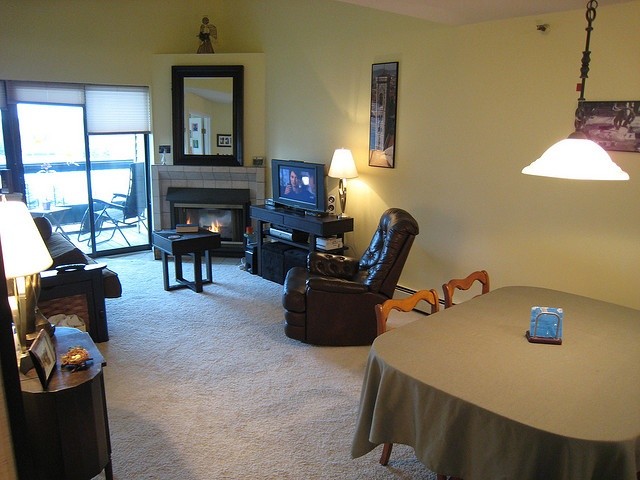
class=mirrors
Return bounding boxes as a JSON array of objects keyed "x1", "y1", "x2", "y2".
[{"x1": 170, "y1": 65, "x2": 244, "y2": 167}]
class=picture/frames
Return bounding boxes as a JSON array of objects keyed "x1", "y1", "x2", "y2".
[
  {"x1": 28, "y1": 328, "x2": 57, "y2": 390},
  {"x1": 217, "y1": 134, "x2": 232, "y2": 147},
  {"x1": 368, "y1": 61, "x2": 399, "y2": 169},
  {"x1": 580, "y1": 101, "x2": 640, "y2": 153}
]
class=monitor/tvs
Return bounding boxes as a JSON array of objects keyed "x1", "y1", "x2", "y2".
[{"x1": 271, "y1": 159, "x2": 325, "y2": 213}]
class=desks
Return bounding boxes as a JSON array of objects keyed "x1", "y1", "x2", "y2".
[{"x1": 29, "y1": 206, "x2": 72, "y2": 240}]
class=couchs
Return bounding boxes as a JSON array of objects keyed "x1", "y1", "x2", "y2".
[
  {"x1": 283, "y1": 207, "x2": 419, "y2": 345},
  {"x1": 1, "y1": 216, "x2": 121, "y2": 344}
]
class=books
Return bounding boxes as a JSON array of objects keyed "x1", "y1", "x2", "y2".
[{"x1": 176, "y1": 223, "x2": 199, "y2": 232}]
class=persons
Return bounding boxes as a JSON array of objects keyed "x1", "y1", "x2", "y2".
[
  {"x1": 283, "y1": 169, "x2": 310, "y2": 201},
  {"x1": 307, "y1": 171, "x2": 317, "y2": 198}
]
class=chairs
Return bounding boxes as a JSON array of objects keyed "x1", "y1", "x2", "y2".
[
  {"x1": 372, "y1": 288, "x2": 440, "y2": 467},
  {"x1": 77, "y1": 162, "x2": 148, "y2": 249},
  {"x1": 442, "y1": 270, "x2": 490, "y2": 312}
]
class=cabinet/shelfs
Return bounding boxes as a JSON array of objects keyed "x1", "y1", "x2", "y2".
[
  {"x1": 249, "y1": 204, "x2": 354, "y2": 287},
  {"x1": 0, "y1": 328, "x2": 109, "y2": 479}
]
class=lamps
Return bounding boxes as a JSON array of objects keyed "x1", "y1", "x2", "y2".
[
  {"x1": 327, "y1": 148, "x2": 358, "y2": 212},
  {"x1": 520, "y1": 0, "x2": 630, "y2": 181},
  {"x1": 0, "y1": 201, "x2": 54, "y2": 374}
]
